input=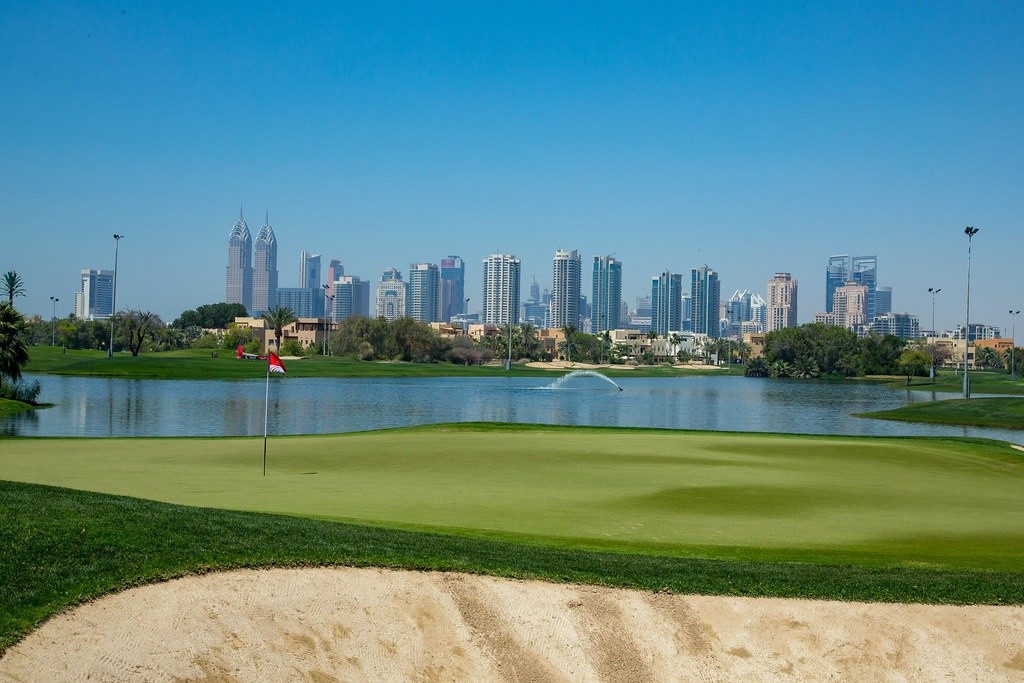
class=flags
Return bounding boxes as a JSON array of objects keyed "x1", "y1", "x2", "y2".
[{"x1": 269, "y1": 352, "x2": 287, "y2": 374}]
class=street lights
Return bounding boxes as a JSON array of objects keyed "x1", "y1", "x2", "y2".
[
  {"x1": 328, "y1": 294, "x2": 335, "y2": 357},
  {"x1": 49, "y1": 297, "x2": 60, "y2": 348},
  {"x1": 964, "y1": 224, "x2": 980, "y2": 400},
  {"x1": 928, "y1": 287, "x2": 942, "y2": 382},
  {"x1": 727, "y1": 309, "x2": 731, "y2": 374},
  {"x1": 322, "y1": 283, "x2": 330, "y2": 357},
  {"x1": 1008, "y1": 309, "x2": 1020, "y2": 374},
  {"x1": 109, "y1": 233, "x2": 123, "y2": 358}
]
